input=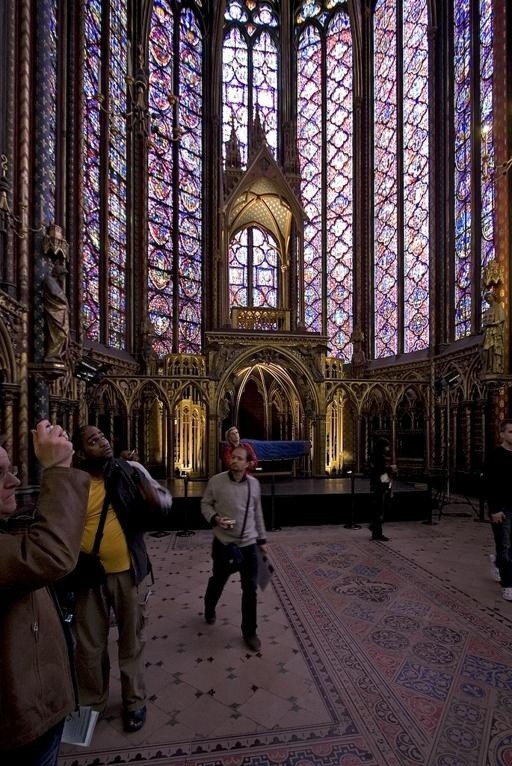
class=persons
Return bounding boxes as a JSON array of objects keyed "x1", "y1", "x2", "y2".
[
  {"x1": 1, "y1": 418, "x2": 93, "y2": 765},
  {"x1": 43, "y1": 264, "x2": 70, "y2": 362},
  {"x1": 200, "y1": 448, "x2": 270, "y2": 651},
  {"x1": 484, "y1": 418, "x2": 512, "y2": 602},
  {"x1": 481, "y1": 291, "x2": 504, "y2": 373},
  {"x1": 54, "y1": 425, "x2": 171, "y2": 734},
  {"x1": 368, "y1": 438, "x2": 398, "y2": 542},
  {"x1": 223, "y1": 426, "x2": 258, "y2": 478}
]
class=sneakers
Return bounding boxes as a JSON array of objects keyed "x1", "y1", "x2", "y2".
[
  {"x1": 500, "y1": 585, "x2": 512, "y2": 600},
  {"x1": 344, "y1": 524, "x2": 362, "y2": 530},
  {"x1": 489, "y1": 554, "x2": 501, "y2": 582},
  {"x1": 372, "y1": 532, "x2": 389, "y2": 541},
  {"x1": 123, "y1": 704, "x2": 146, "y2": 732},
  {"x1": 205, "y1": 605, "x2": 216, "y2": 624},
  {"x1": 242, "y1": 627, "x2": 260, "y2": 650}
]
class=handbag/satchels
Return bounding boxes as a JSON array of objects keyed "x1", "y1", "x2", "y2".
[
  {"x1": 215, "y1": 542, "x2": 241, "y2": 576},
  {"x1": 47, "y1": 551, "x2": 105, "y2": 595},
  {"x1": 127, "y1": 458, "x2": 172, "y2": 511}
]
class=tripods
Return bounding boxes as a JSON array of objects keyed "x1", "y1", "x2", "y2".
[{"x1": 437, "y1": 473, "x2": 479, "y2": 520}]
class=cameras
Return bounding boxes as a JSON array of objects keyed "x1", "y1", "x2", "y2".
[
  {"x1": 223, "y1": 518, "x2": 237, "y2": 528},
  {"x1": 46, "y1": 426, "x2": 71, "y2": 440}
]
